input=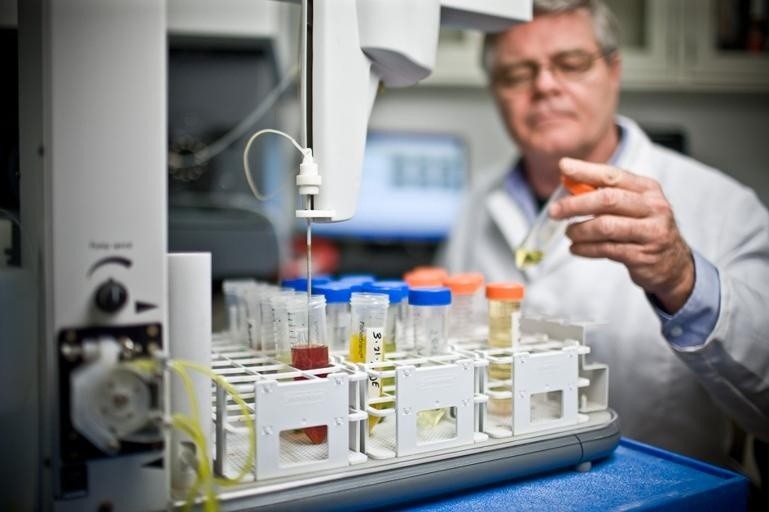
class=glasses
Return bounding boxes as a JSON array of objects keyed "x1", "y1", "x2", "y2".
[{"x1": 487, "y1": 42, "x2": 620, "y2": 97}]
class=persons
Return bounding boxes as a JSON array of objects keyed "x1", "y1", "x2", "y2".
[{"x1": 418, "y1": 0, "x2": 769, "y2": 482}]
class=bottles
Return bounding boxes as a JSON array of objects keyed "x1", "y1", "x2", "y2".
[
  {"x1": 509, "y1": 171, "x2": 598, "y2": 271},
  {"x1": 221, "y1": 269, "x2": 525, "y2": 439}
]
